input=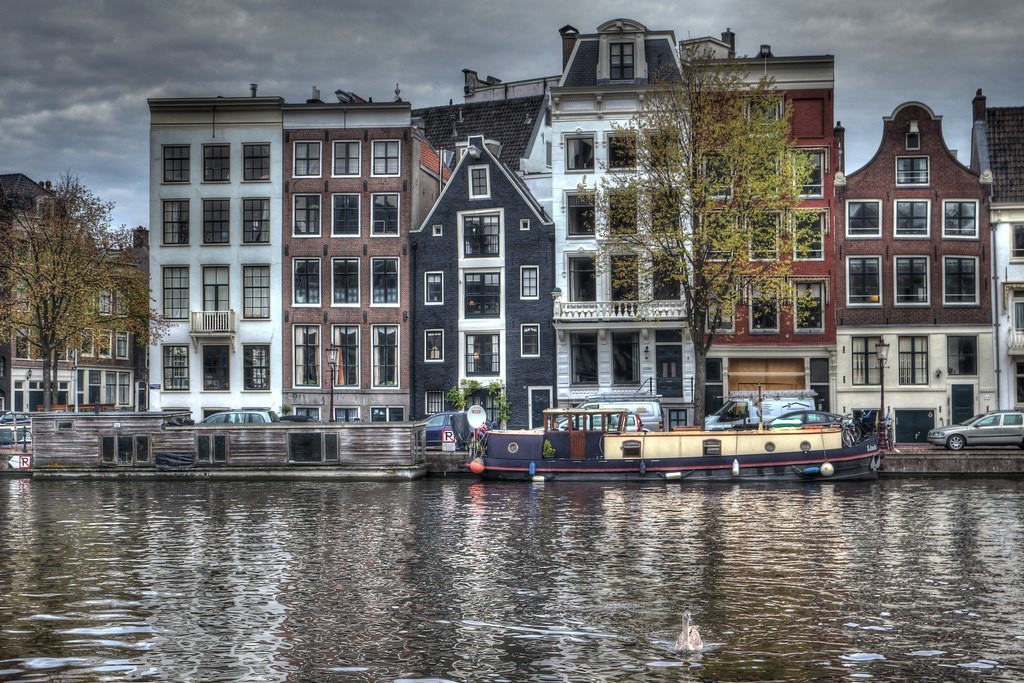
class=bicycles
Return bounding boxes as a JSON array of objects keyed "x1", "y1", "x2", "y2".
[{"x1": 833, "y1": 414, "x2": 895, "y2": 451}]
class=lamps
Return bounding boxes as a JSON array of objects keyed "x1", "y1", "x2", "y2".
[{"x1": 27, "y1": 368, "x2": 32, "y2": 380}]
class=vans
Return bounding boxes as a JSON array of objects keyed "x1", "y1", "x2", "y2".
[
  {"x1": 552, "y1": 393, "x2": 665, "y2": 433},
  {"x1": 705, "y1": 390, "x2": 818, "y2": 429}
]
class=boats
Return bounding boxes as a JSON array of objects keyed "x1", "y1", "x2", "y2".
[{"x1": 460, "y1": 381, "x2": 886, "y2": 483}]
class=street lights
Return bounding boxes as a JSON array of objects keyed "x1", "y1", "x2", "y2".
[
  {"x1": 324, "y1": 343, "x2": 341, "y2": 422},
  {"x1": 875, "y1": 339, "x2": 890, "y2": 449}
]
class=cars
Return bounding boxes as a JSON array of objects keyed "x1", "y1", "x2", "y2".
[
  {"x1": 764, "y1": 409, "x2": 854, "y2": 427},
  {"x1": 414, "y1": 411, "x2": 500, "y2": 449},
  {"x1": 0, "y1": 409, "x2": 30, "y2": 442},
  {"x1": 196, "y1": 406, "x2": 281, "y2": 423},
  {"x1": 555, "y1": 412, "x2": 644, "y2": 431},
  {"x1": 926, "y1": 408, "x2": 1024, "y2": 450}
]
